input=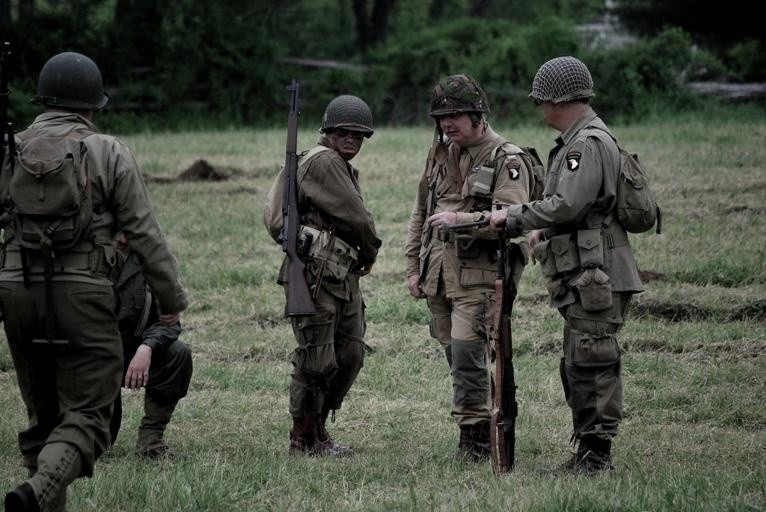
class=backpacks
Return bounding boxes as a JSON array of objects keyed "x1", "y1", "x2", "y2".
[
  {"x1": 522, "y1": 146, "x2": 547, "y2": 200},
  {"x1": 262, "y1": 165, "x2": 289, "y2": 245},
  {"x1": 8, "y1": 136, "x2": 93, "y2": 253},
  {"x1": 615, "y1": 152, "x2": 657, "y2": 234}
]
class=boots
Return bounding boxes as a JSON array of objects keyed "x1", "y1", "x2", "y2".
[
  {"x1": 559, "y1": 437, "x2": 583, "y2": 470},
  {"x1": 4, "y1": 440, "x2": 84, "y2": 512},
  {"x1": 315, "y1": 407, "x2": 353, "y2": 450},
  {"x1": 134, "y1": 401, "x2": 187, "y2": 461},
  {"x1": 288, "y1": 416, "x2": 354, "y2": 457},
  {"x1": 571, "y1": 438, "x2": 612, "y2": 476},
  {"x1": 23, "y1": 453, "x2": 67, "y2": 512},
  {"x1": 456, "y1": 425, "x2": 490, "y2": 460}
]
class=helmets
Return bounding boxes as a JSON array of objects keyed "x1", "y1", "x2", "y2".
[
  {"x1": 529, "y1": 56, "x2": 594, "y2": 104},
  {"x1": 319, "y1": 95, "x2": 374, "y2": 138},
  {"x1": 31, "y1": 51, "x2": 109, "y2": 111},
  {"x1": 427, "y1": 75, "x2": 491, "y2": 117}
]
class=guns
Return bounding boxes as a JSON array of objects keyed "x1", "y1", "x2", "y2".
[
  {"x1": 281, "y1": 78, "x2": 318, "y2": 317},
  {"x1": 1, "y1": 41, "x2": 15, "y2": 177},
  {"x1": 488, "y1": 204, "x2": 515, "y2": 472}
]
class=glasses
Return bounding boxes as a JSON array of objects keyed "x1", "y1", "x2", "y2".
[{"x1": 333, "y1": 128, "x2": 364, "y2": 140}]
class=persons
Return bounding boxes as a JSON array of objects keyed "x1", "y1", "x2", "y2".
[
  {"x1": 275, "y1": 95, "x2": 382, "y2": 458},
  {"x1": 114, "y1": 229, "x2": 194, "y2": 461},
  {"x1": 402, "y1": 72, "x2": 536, "y2": 466},
  {"x1": 0, "y1": 52, "x2": 192, "y2": 511},
  {"x1": 426, "y1": 55, "x2": 646, "y2": 479}
]
